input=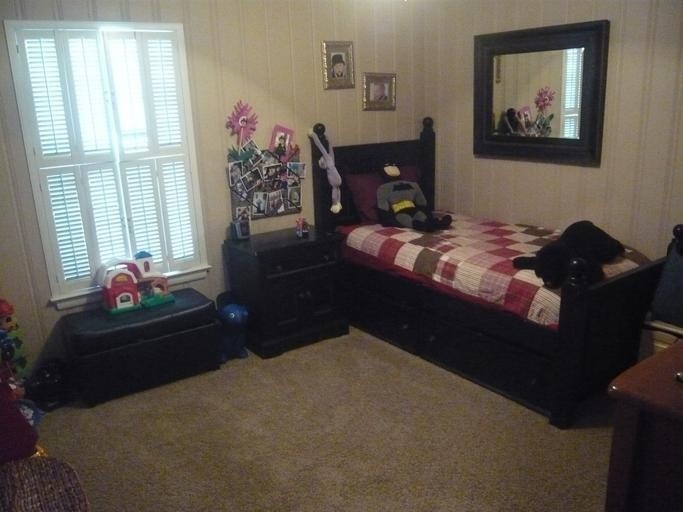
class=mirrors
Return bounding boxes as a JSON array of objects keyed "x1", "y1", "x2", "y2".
[{"x1": 472, "y1": 18, "x2": 610, "y2": 168}]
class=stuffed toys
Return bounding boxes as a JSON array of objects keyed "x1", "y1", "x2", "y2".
[
  {"x1": 307, "y1": 128, "x2": 343, "y2": 215},
  {"x1": 511, "y1": 219, "x2": 625, "y2": 288}
]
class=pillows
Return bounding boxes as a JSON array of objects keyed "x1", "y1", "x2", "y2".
[{"x1": 342, "y1": 163, "x2": 424, "y2": 224}]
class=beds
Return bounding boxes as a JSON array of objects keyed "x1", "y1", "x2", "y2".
[{"x1": 307, "y1": 116, "x2": 682, "y2": 430}]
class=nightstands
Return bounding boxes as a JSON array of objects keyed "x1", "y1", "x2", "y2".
[{"x1": 224, "y1": 225, "x2": 351, "y2": 360}]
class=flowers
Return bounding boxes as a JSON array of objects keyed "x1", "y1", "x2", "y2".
[{"x1": 533, "y1": 86, "x2": 558, "y2": 136}]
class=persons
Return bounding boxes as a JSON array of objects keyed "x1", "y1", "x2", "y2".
[
  {"x1": 376, "y1": 162, "x2": 453, "y2": 230},
  {"x1": 330, "y1": 54, "x2": 345, "y2": 79},
  {"x1": 230, "y1": 134, "x2": 305, "y2": 216},
  {"x1": 373, "y1": 83, "x2": 388, "y2": 102}
]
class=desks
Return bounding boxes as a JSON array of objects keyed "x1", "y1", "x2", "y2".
[
  {"x1": 604, "y1": 335, "x2": 683, "y2": 511},
  {"x1": 59, "y1": 285, "x2": 222, "y2": 407}
]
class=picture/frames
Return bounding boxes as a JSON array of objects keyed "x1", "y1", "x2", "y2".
[
  {"x1": 324, "y1": 40, "x2": 356, "y2": 90},
  {"x1": 363, "y1": 71, "x2": 397, "y2": 112}
]
